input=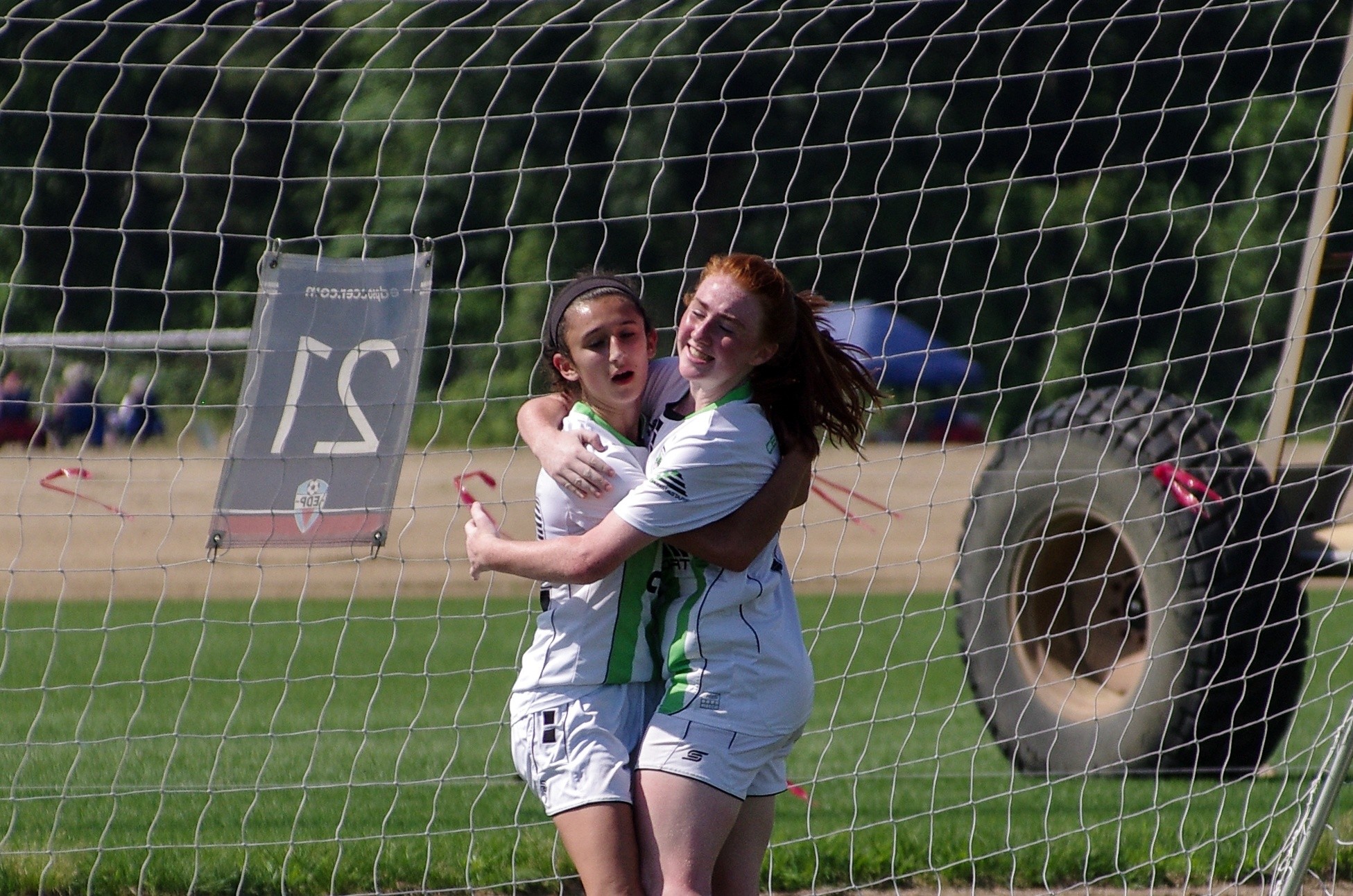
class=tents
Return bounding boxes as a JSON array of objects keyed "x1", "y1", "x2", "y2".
[{"x1": 810, "y1": 299, "x2": 984, "y2": 384}]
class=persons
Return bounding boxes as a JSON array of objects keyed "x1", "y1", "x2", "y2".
[
  {"x1": 509, "y1": 268, "x2": 811, "y2": 896},
  {"x1": 49, "y1": 361, "x2": 106, "y2": 447},
  {"x1": 111, "y1": 372, "x2": 165, "y2": 443},
  {"x1": 466, "y1": 256, "x2": 880, "y2": 896},
  {"x1": 0, "y1": 372, "x2": 47, "y2": 447}
]
